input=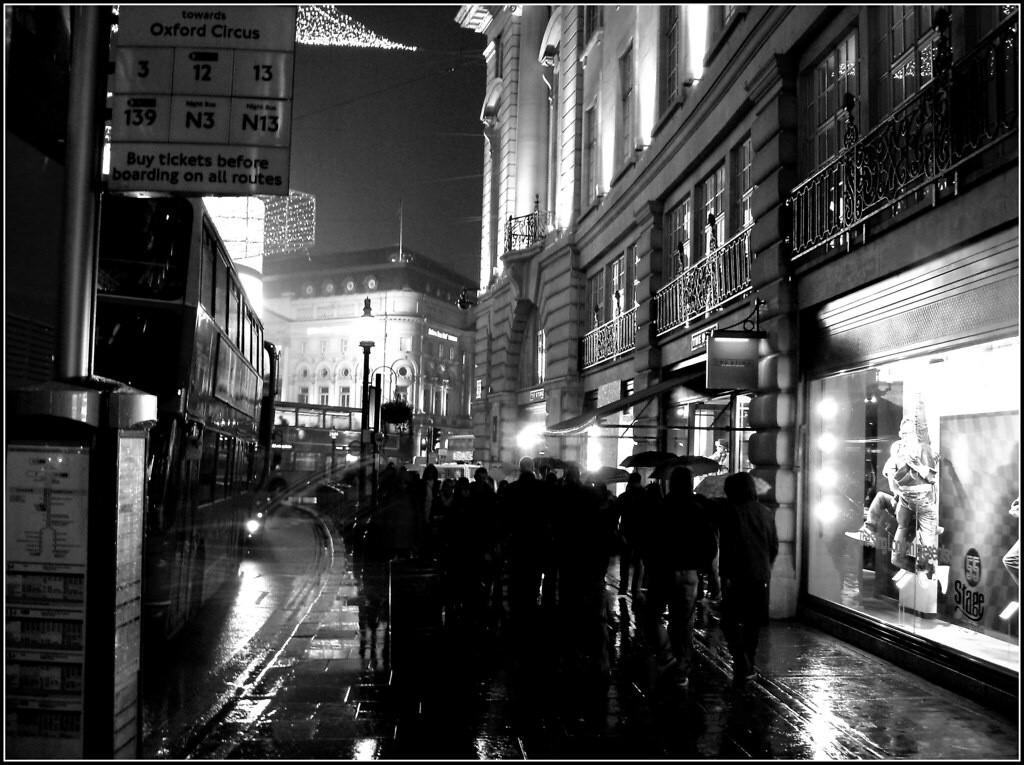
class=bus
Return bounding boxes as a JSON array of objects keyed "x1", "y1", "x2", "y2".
[{"x1": 9, "y1": 193, "x2": 279, "y2": 636}]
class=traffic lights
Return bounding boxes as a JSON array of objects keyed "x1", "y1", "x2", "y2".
[{"x1": 432, "y1": 427, "x2": 441, "y2": 452}]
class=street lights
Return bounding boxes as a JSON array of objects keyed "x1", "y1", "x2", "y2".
[{"x1": 357, "y1": 296, "x2": 377, "y2": 498}]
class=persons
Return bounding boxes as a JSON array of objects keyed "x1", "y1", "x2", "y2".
[
  {"x1": 845, "y1": 416, "x2": 944, "y2": 547},
  {"x1": 891, "y1": 381, "x2": 937, "y2": 573},
  {"x1": 646, "y1": 468, "x2": 717, "y2": 670},
  {"x1": 719, "y1": 472, "x2": 779, "y2": 679},
  {"x1": 345, "y1": 457, "x2": 732, "y2": 674},
  {"x1": 617, "y1": 472, "x2": 647, "y2": 595},
  {"x1": 1002, "y1": 496, "x2": 1019, "y2": 586}
]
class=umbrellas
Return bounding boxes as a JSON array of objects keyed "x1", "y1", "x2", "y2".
[
  {"x1": 580, "y1": 466, "x2": 630, "y2": 483},
  {"x1": 648, "y1": 455, "x2": 721, "y2": 479},
  {"x1": 619, "y1": 451, "x2": 679, "y2": 467},
  {"x1": 533, "y1": 458, "x2": 569, "y2": 479},
  {"x1": 693, "y1": 472, "x2": 772, "y2": 499}
]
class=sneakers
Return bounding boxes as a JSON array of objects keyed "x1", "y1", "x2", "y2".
[
  {"x1": 845, "y1": 529, "x2": 875, "y2": 547},
  {"x1": 938, "y1": 566, "x2": 950, "y2": 594}
]
